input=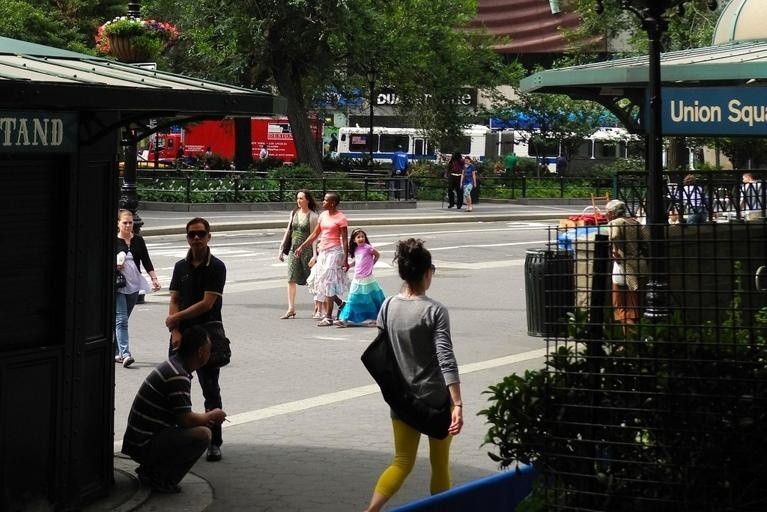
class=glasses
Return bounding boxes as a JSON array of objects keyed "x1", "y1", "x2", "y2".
[
  {"x1": 427, "y1": 263, "x2": 436, "y2": 274},
  {"x1": 187, "y1": 229, "x2": 207, "y2": 238}
]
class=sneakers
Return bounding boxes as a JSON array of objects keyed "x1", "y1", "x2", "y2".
[
  {"x1": 313, "y1": 313, "x2": 320, "y2": 318},
  {"x1": 115, "y1": 354, "x2": 122, "y2": 362},
  {"x1": 122, "y1": 353, "x2": 134, "y2": 367}
]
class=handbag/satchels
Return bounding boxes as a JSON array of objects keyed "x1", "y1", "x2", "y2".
[
  {"x1": 360, "y1": 326, "x2": 451, "y2": 440},
  {"x1": 116, "y1": 272, "x2": 126, "y2": 289},
  {"x1": 205, "y1": 330, "x2": 231, "y2": 367},
  {"x1": 282, "y1": 230, "x2": 293, "y2": 255}
]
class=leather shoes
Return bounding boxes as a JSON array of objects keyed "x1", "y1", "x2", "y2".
[
  {"x1": 456, "y1": 205, "x2": 461, "y2": 209},
  {"x1": 205, "y1": 445, "x2": 221, "y2": 461},
  {"x1": 448, "y1": 204, "x2": 453, "y2": 208},
  {"x1": 139, "y1": 472, "x2": 180, "y2": 493}
]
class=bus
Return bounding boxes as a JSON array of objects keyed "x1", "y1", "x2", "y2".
[{"x1": 338, "y1": 127, "x2": 667, "y2": 176}]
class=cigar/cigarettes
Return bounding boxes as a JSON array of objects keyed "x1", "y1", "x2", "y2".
[{"x1": 225, "y1": 418, "x2": 231, "y2": 423}]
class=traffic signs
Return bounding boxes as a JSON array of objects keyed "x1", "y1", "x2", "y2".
[{"x1": 661, "y1": 85, "x2": 767, "y2": 136}]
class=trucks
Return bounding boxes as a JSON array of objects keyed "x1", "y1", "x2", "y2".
[{"x1": 147, "y1": 118, "x2": 325, "y2": 165}]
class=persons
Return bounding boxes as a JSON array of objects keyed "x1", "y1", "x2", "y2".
[
  {"x1": 460, "y1": 156, "x2": 477, "y2": 212},
  {"x1": 738, "y1": 172, "x2": 767, "y2": 224},
  {"x1": 178, "y1": 142, "x2": 185, "y2": 159},
  {"x1": 329, "y1": 133, "x2": 338, "y2": 152},
  {"x1": 293, "y1": 191, "x2": 350, "y2": 326},
  {"x1": 332, "y1": 228, "x2": 384, "y2": 328},
  {"x1": 361, "y1": 239, "x2": 463, "y2": 512},
  {"x1": 389, "y1": 145, "x2": 408, "y2": 201},
  {"x1": 280, "y1": 189, "x2": 319, "y2": 320},
  {"x1": 259, "y1": 145, "x2": 269, "y2": 159},
  {"x1": 556, "y1": 153, "x2": 568, "y2": 177},
  {"x1": 121, "y1": 324, "x2": 226, "y2": 493},
  {"x1": 205, "y1": 147, "x2": 213, "y2": 157},
  {"x1": 444, "y1": 152, "x2": 464, "y2": 209},
  {"x1": 117, "y1": 208, "x2": 161, "y2": 367},
  {"x1": 672, "y1": 174, "x2": 707, "y2": 223},
  {"x1": 604, "y1": 199, "x2": 642, "y2": 337},
  {"x1": 307, "y1": 240, "x2": 328, "y2": 319},
  {"x1": 166, "y1": 218, "x2": 226, "y2": 461}
]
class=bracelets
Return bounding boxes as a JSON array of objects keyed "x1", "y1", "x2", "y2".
[
  {"x1": 454, "y1": 401, "x2": 462, "y2": 407},
  {"x1": 151, "y1": 276, "x2": 157, "y2": 281}
]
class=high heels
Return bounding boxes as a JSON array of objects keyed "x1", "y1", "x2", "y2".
[{"x1": 280, "y1": 311, "x2": 295, "y2": 319}]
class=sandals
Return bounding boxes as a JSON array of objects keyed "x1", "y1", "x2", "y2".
[
  {"x1": 317, "y1": 317, "x2": 333, "y2": 326},
  {"x1": 333, "y1": 320, "x2": 346, "y2": 328},
  {"x1": 366, "y1": 321, "x2": 376, "y2": 326}
]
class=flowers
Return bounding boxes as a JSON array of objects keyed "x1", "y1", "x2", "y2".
[{"x1": 95, "y1": 15, "x2": 180, "y2": 51}]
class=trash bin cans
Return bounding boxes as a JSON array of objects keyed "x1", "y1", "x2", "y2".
[{"x1": 525, "y1": 249, "x2": 575, "y2": 337}]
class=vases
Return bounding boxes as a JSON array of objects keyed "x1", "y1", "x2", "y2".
[{"x1": 108, "y1": 35, "x2": 148, "y2": 63}]
